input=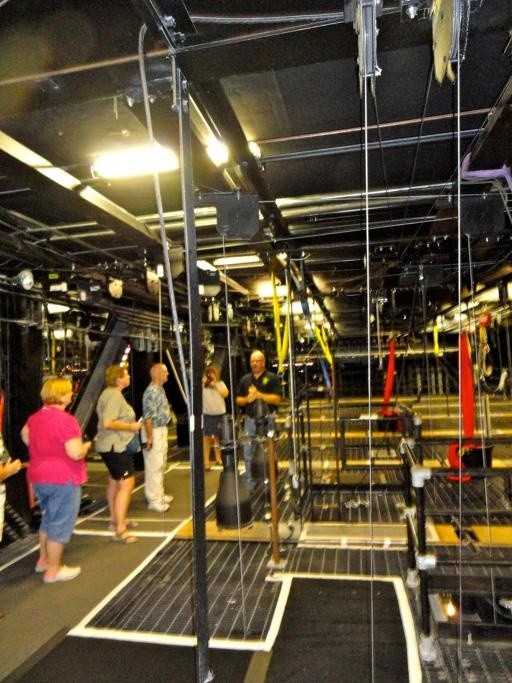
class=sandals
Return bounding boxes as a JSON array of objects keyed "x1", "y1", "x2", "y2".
[
  {"x1": 34, "y1": 559, "x2": 48, "y2": 572},
  {"x1": 107, "y1": 521, "x2": 136, "y2": 530},
  {"x1": 43, "y1": 565, "x2": 82, "y2": 584},
  {"x1": 112, "y1": 531, "x2": 137, "y2": 544}
]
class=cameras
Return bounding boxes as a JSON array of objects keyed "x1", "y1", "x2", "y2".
[{"x1": 206, "y1": 373, "x2": 213, "y2": 382}]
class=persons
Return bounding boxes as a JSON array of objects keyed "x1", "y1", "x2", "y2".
[
  {"x1": 202, "y1": 365, "x2": 229, "y2": 471},
  {"x1": 18, "y1": 376, "x2": 91, "y2": 584},
  {"x1": 139, "y1": 364, "x2": 174, "y2": 513},
  {"x1": 92, "y1": 364, "x2": 144, "y2": 544},
  {"x1": 235, "y1": 349, "x2": 282, "y2": 488}
]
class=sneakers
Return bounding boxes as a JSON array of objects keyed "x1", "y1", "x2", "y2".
[
  {"x1": 162, "y1": 494, "x2": 174, "y2": 504},
  {"x1": 148, "y1": 500, "x2": 169, "y2": 512}
]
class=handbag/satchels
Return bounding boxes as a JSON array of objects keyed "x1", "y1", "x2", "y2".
[{"x1": 127, "y1": 434, "x2": 141, "y2": 455}]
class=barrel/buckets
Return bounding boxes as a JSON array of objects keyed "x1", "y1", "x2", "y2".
[{"x1": 464, "y1": 441, "x2": 493, "y2": 469}]
